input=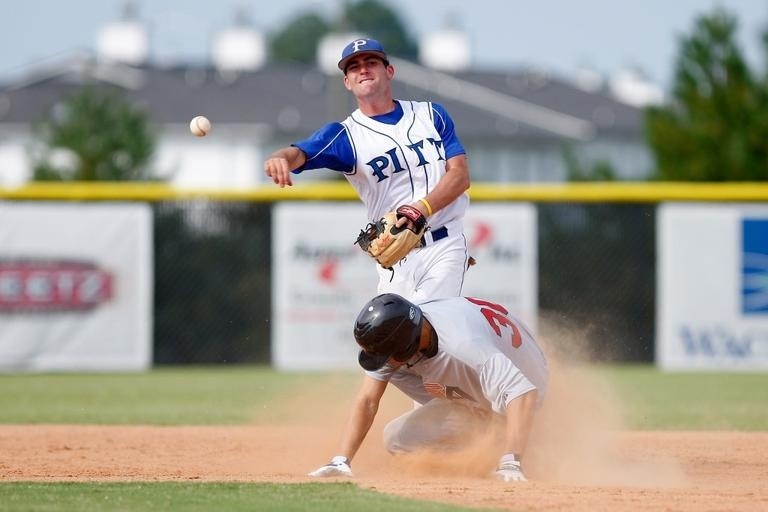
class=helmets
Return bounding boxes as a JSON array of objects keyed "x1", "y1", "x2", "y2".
[{"x1": 354, "y1": 293, "x2": 423, "y2": 371}]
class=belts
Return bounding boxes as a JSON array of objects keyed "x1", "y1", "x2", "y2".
[{"x1": 410, "y1": 227, "x2": 447, "y2": 250}]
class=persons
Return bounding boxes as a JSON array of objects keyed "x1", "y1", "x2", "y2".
[
  {"x1": 307, "y1": 292, "x2": 548, "y2": 482},
  {"x1": 262, "y1": 37, "x2": 472, "y2": 405}
]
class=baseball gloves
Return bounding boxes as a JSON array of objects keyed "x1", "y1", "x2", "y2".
[{"x1": 357, "y1": 206, "x2": 428, "y2": 268}]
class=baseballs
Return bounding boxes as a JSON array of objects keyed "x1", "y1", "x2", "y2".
[{"x1": 189, "y1": 116, "x2": 211, "y2": 135}]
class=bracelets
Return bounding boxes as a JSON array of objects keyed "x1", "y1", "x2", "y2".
[{"x1": 420, "y1": 198, "x2": 432, "y2": 217}]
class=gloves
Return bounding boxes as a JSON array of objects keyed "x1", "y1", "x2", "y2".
[
  {"x1": 496, "y1": 453, "x2": 529, "y2": 483},
  {"x1": 307, "y1": 456, "x2": 353, "y2": 478}
]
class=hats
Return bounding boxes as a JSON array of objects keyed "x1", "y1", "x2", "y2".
[{"x1": 338, "y1": 38, "x2": 390, "y2": 70}]
